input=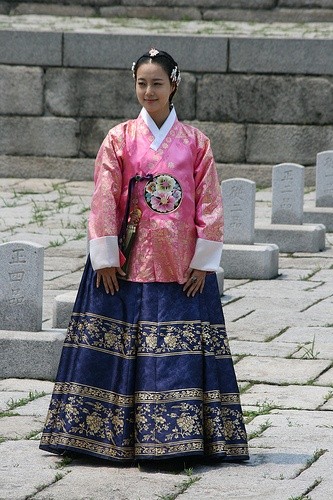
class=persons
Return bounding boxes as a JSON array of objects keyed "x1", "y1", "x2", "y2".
[{"x1": 40, "y1": 48, "x2": 251, "y2": 460}]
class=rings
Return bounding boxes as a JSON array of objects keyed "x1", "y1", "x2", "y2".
[{"x1": 190, "y1": 276, "x2": 196, "y2": 283}]
